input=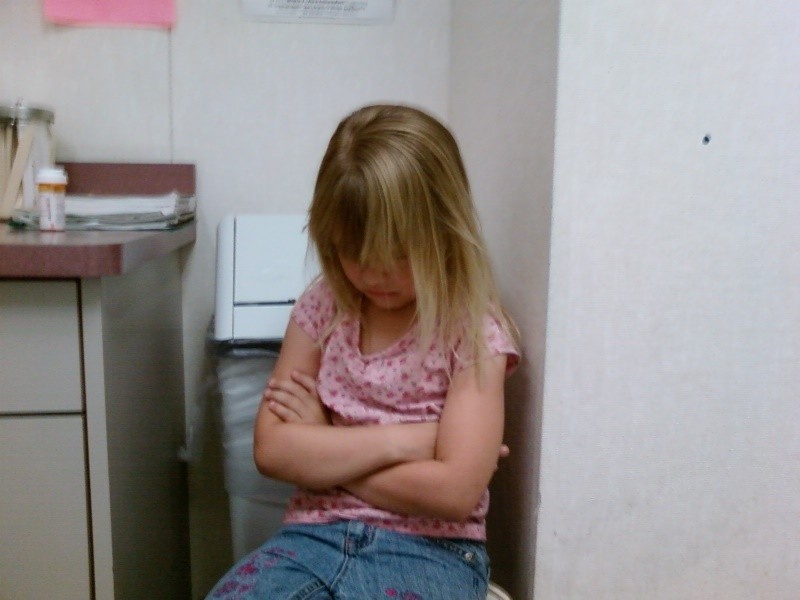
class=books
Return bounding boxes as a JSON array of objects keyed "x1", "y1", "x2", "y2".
[{"x1": 64, "y1": 191, "x2": 196, "y2": 230}]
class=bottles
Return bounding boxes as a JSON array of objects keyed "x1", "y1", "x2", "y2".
[
  {"x1": 0, "y1": 106, "x2": 55, "y2": 222},
  {"x1": 35, "y1": 167, "x2": 69, "y2": 232}
]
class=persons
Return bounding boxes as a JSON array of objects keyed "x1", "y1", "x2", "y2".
[{"x1": 206, "y1": 104, "x2": 522, "y2": 600}]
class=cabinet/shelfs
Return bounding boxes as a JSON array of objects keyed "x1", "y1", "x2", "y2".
[{"x1": 0, "y1": 251, "x2": 192, "y2": 600}]
